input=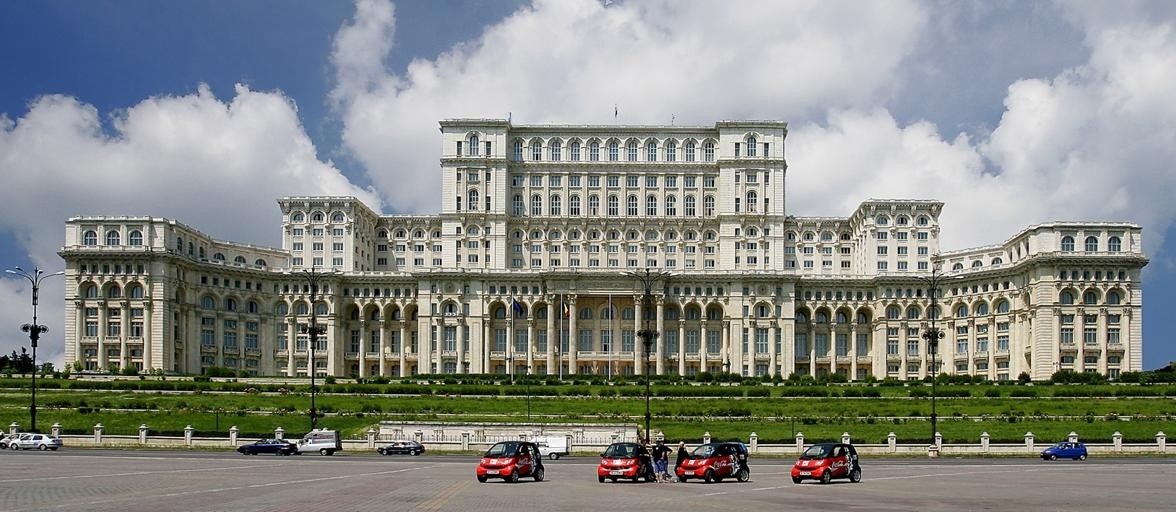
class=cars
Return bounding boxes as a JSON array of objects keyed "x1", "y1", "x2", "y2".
[
  {"x1": 676, "y1": 439, "x2": 751, "y2": 483},
  {"x1": 791, "y1": 443, "x2": 861, "y2": 484},
  {"x1": 1039, "y1": 441, "x2": 1087, "y2": 462},
  {"x1": 7, "y1": 433, "x2": 63, "y2": 451},
  {"x1": 475, "y1": 439, "x2": 544, "y2": 484},
  {"x1": 723, "y1": 441, "x2": 749, "y2": 458},
  {"x1": 376, "y1": 439, "x2": 424, "y2": 457},
  {"x1": 234, "y1": 436, "x2": 297, "y2": 457},
  {"x1": 0, "y1": 432, "x2": 31, "y2": 450},
  {"x1": 595, "y1": 441, "x2": 655, "y2": 483}
]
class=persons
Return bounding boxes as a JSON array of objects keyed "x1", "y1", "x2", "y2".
[
  {"x1": 637, "y1": 435, "x2": 673, "y2": 484},
  {"x1": 674, "y1": 441, "x2": 689, "y2": 475}
]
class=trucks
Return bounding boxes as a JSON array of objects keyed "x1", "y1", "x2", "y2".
[
  {"x1": 294, "y1": 428, "x2": 342, "y2": 456},
  {"x1": 521, "y1": 435, "x2": 572, "y2": 460}
]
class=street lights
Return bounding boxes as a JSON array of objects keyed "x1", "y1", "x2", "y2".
[
  {"x1": 620, "y1": 266, "x2": 672, "y2": 442},
  {"x1": 913, "y1": 262, "x2": 963, "y2": 447},
  {"x1": 278, "y1": 266, "x2": 342, "y2": 430},
  {"x1": 4, "y1": 264, "x2": 66, "y2": 433}
]
